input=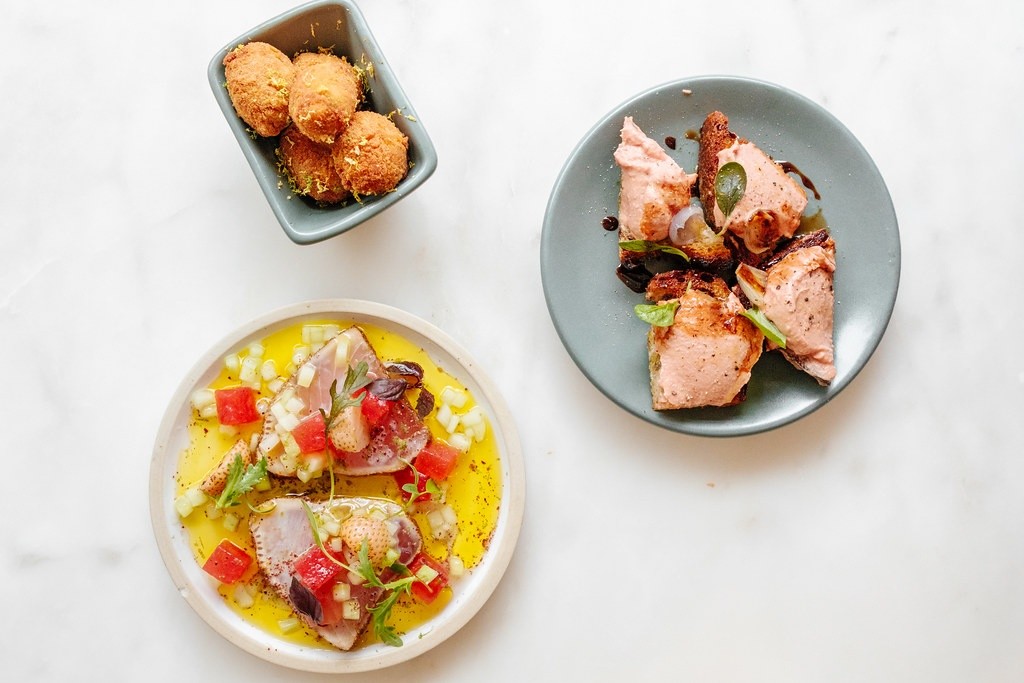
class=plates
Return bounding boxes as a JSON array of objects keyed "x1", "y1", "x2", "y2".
[{"x1": 539, "y1": 76, "x2": 902, "y2": 439}]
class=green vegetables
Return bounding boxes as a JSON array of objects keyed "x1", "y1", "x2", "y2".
[
  {"x1": 619, "y1": 163, "x2": 788, "y2": 349},
  {"x1": 208, "y1": 359, "x2": 444, "y2": 648}
]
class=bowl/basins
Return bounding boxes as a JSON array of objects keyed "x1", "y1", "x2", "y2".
[
  {"x1": 149, "y1": 297, "x2": 527, "y2": 675},
  {"x1": 206, "y1": 0, "x2": 438, "y2": 250}
]
